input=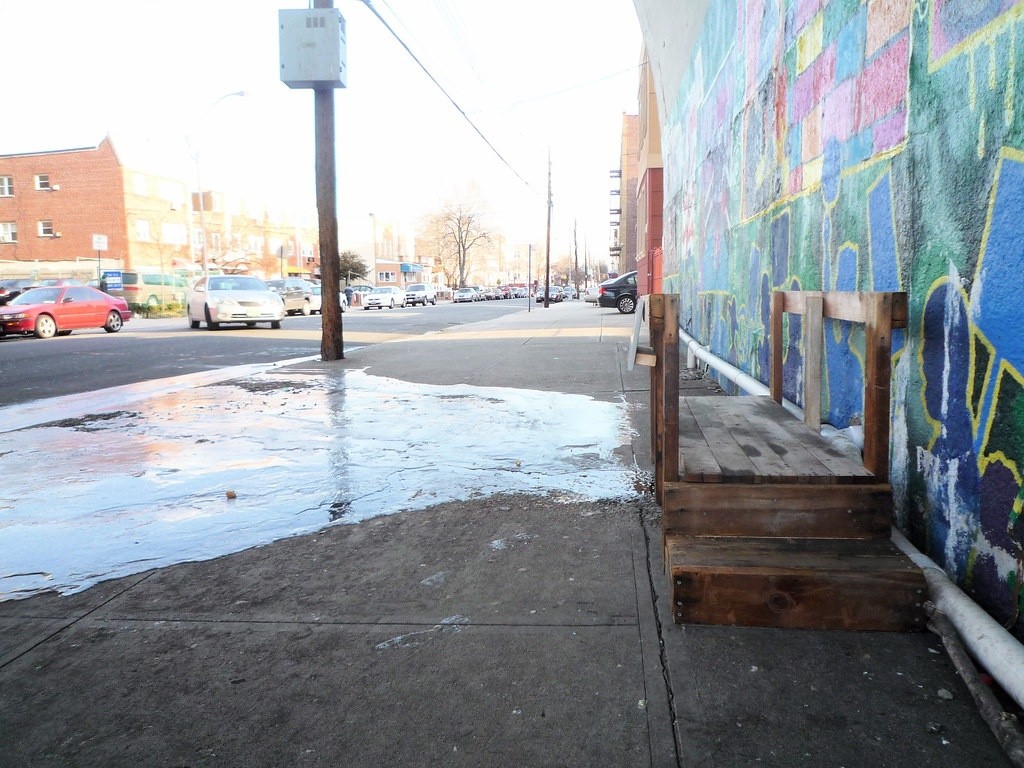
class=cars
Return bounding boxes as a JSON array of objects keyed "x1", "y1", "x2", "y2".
[
  {"x1": 266, "y1": 275, "x2": 348, "y2": 316},
  {"x1": 584, "y1": 278, "x2": 617, "y2": 302},
  {"x1": 472, "y1": 284, "x2": 535, "y2": 302},
  {"x1": 0, "y1": 285, "x2": 132, "y2": 340},
  {"x1": 0, "y1": 278, "x2": 96, "y2": 306},
  {"x1": 453, "y1": 287, "x2": 479, "y2": 303},
  {"x1": 187, "y1": 274, "x2": 286, "y2": 331},
  {"x1": 536, "y1": 286, "x2": 563, "y2": 303},
  {"x1": 598, "y1": 271, "x2": 639, "y2": 314},
  {"x1": 350, "y1": 284, "x2": 374, "y2": 295},
  {"x1": 361, "y1": 285, "x2": 408, "y2": 310},
  {"x1": 558, "y1": 285, "x2": 577, "y2": 300}
]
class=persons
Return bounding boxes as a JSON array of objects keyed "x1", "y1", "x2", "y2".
[
  {"x1": 345, "y1": 283, "x2": 354, "y2": 306},
  {"x1": 0, "y1": 287, "x2": 10, "y2": 306}
]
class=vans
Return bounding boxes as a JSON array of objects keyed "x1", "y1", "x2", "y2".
[{"x1": 109, "y1": 268, "x2": 189, "y2": 311}]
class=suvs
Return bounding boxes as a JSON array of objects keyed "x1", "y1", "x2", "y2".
[{"x1": 404, "y1": 283, "x2": 438, "y2": 307}]
